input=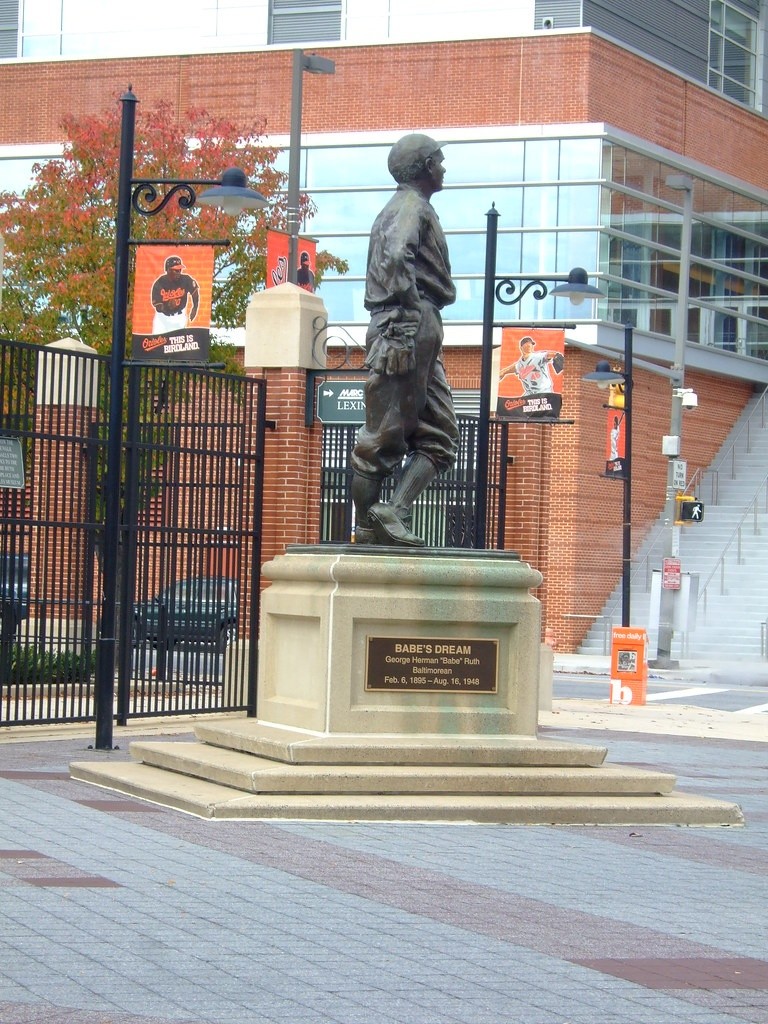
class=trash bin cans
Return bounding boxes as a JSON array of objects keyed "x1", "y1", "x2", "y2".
[{"x1": 610, "y1": 625, "x2": 651, "y2": 705}]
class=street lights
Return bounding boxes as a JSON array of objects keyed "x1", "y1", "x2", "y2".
[
  {"x1": 655, "y1": 174, "x2": 698, "y2": 658},
  {"x1": 471, "y1": 205, "x2": 609, "y2": 550},
  {"x1": 581, "y1": 326, "x2": 636, "y2": 627},
  {"x1": 90, "y1": 80, "x2": 272, "y2": 751},
  {"x1": 282, "y1": 48, "x2": 335, "y2": 283}
]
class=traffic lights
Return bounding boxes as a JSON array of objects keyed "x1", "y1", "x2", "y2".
[{"x1": 681, "y1": 501, "x2": 704, "y2": 523}]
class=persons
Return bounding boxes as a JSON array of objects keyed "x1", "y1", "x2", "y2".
[
  {"x1": 297, "y1": 251, "x2": 313, "y2": 293},
  {"x1": 352, "y1": 133, "x2": 460, "y2": 547},
  {"x1": 150, "y1": 256, "x2": 198, "y2": 335},
  {"x1": 608, "y1": 412, "x2": 625, "y2": 461},
  {"x1": 500, "y1": 336, "x2": 564, "y2": 395}
]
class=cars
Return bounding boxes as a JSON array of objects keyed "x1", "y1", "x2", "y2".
[
  {"x1": 134, "y1": 575, "x2": 237, "y2": 655},
  {"x1": 0, "y1": 553, "x2": 30, "y2": 621}
]
